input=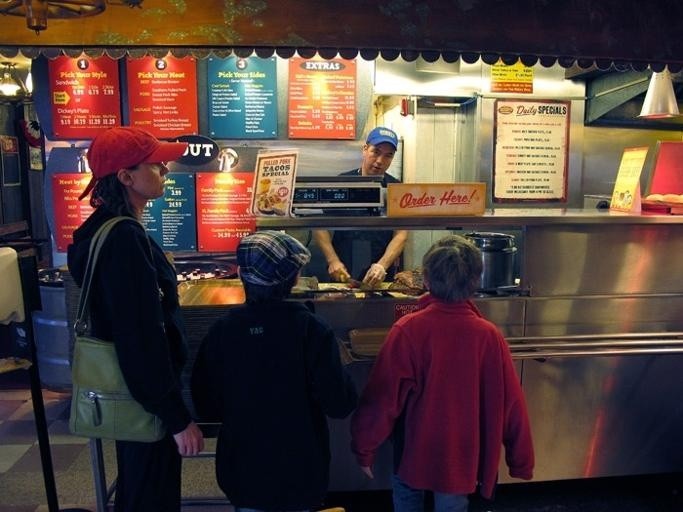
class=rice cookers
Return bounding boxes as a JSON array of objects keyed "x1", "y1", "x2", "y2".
[{"x1": 464, "y1": 232, "x2": 517, "y2": 294}]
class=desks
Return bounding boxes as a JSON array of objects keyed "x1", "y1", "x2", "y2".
[{"x1": 0, "y1": 246, "x2": 25, "y2": 328}]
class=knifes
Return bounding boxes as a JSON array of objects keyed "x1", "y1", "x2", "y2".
[{"x1": 328, "y1": 266, "x2": 373, "y2": 293}]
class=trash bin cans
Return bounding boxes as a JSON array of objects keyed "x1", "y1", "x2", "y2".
[{"x1": 33, "y1": 267, "x2": 74, "y2": 391}]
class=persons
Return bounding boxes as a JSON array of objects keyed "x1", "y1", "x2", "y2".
[
  {"x1": 349, "y1": 235, "x2": 535, "y2": 511},
  {"x1": 185, "y1": 228, "x2": 354, "y2": 512},
  {"x1": 65, "y1": 127, "x2": 204, "y2": 512},
  {"x1": 309, "y1": 127, "x2": 410, "y2": 287}
]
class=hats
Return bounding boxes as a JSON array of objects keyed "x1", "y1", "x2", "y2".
[
  {"x1": 237, "y1": 230, "x2": 312, "y2": 285},
  {"x1": 366, "y1": 127, "x2": 398, "y2": 151},
  {"x1": 88, "y1": 127, "x2": 188, "y2": 179}
]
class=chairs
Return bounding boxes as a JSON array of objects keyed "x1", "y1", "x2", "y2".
[{"x1": 0, "y1": 228, "x2": 66, "y2": 512}]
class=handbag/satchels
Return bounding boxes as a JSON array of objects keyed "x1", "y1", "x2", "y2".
[{"x1": 70, "y1": 337, "x2": 168, "y2": 443}]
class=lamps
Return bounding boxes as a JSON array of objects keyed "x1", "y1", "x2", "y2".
[
  {"x1": 0, "y1": 61, "x2": 33, "y2": 101},
  {"x1": 75, "y1": 147, "x2": 88, "y2": 172},
  {"x1": 213, "y1": 147, "x2": 236, "y2": 172},
  {"x1": 0, "y1": 0, "x2": 108, "y2": 38},
  {"x1": 636, "y1": 62, "x2": 682, "y2": 119}
]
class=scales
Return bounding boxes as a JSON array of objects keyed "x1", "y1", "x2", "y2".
[{"x1": 291, "y1": 175, "x2": 388, "y2": 218}]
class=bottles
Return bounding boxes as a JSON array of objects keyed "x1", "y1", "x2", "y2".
[{"x1": 180, "y1": 267, "x2": 224, "y2": 280}]
